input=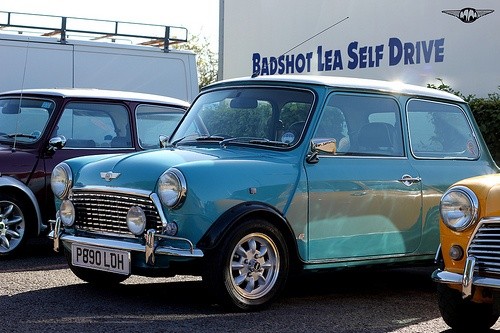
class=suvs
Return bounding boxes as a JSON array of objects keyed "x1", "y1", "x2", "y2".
[
  {"x1": 0, "y1": 88, "x2": 192, "y2": 259},
  {"x1": 431, "y1": 174, "x2": 500, "y2": 333},
  {"x1": 47, "y1": 75, "x2": 499, "y2": 313}
]
